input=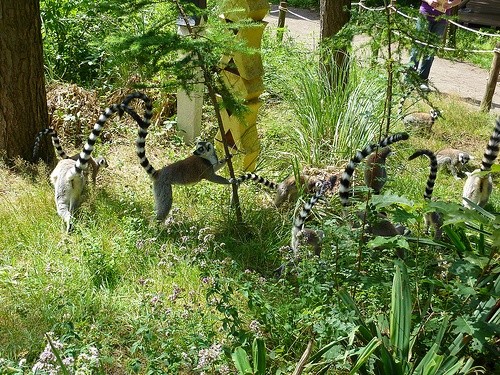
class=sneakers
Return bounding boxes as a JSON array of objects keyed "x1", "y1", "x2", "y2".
[{"x1": 418, "y1": 81, "x2": 430, "y2": 91}]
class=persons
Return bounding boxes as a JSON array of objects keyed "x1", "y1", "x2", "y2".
[{"x1": 402, "y1": 0, "x2": 462, "y2": 92}]
